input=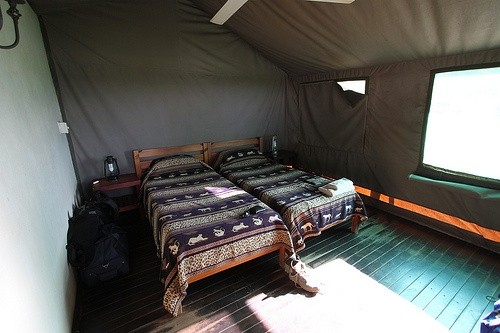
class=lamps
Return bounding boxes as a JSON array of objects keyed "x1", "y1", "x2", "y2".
[
  {"x1": 0, "y1": 0, "x2": 27, "y2": 49},
  {"x1": 104, "y1": 156, "x2": 120, "y2": 183}
]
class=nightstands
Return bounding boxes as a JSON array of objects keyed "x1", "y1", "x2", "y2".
[{"x1": 89, "y1": 173, "x2": 141, "y2": 212}]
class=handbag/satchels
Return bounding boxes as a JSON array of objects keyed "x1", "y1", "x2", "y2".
[{"x1": 69, "y1": 206, "x2": 136, "y2": 284}]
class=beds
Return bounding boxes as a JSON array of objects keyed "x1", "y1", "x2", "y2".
[
  {"x1": 132, "y1": 143, "x2": 318, "y2": 317},
  {"x1": 208, "y1": 136, "x2": 368, "y2": 260}
]
